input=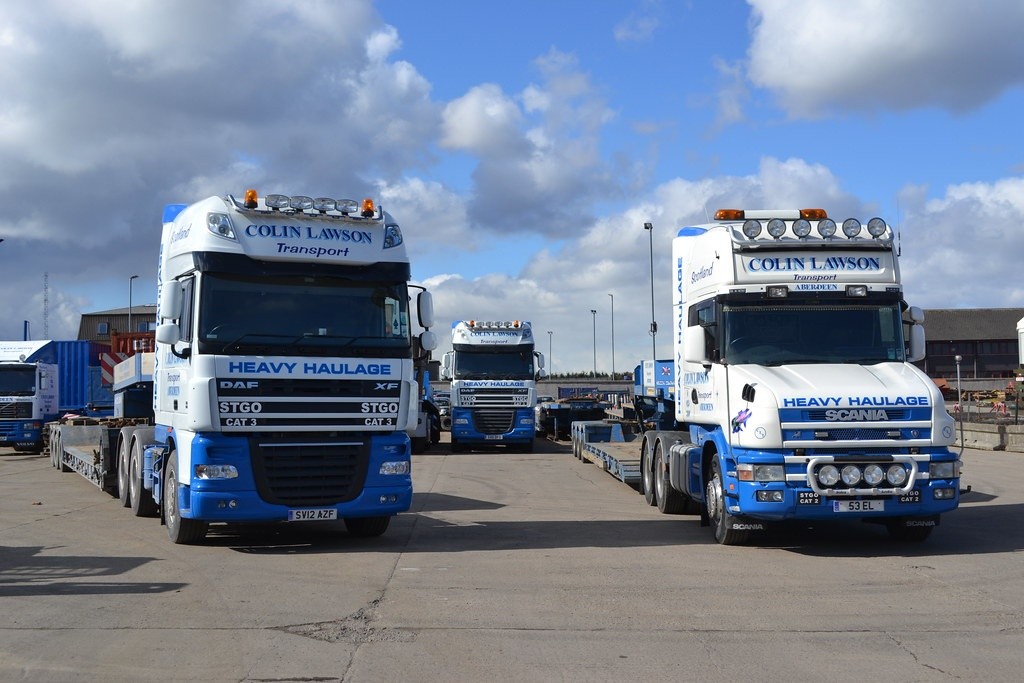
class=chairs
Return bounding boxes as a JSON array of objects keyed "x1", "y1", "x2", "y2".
[{"x1": 304, "y1": 295, "x2": 350, "y2": 337}]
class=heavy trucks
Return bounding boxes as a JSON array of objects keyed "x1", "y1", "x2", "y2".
[
  {"x1": 441, "y1": 320, "x2": 546, "y2": 452},
  {"x1": 50, "y1": 189, "x2": 438, "y2": 545},
  {"x1": 571, "y1": 209, "x2": 964, "y2": 545},
  {"x1": 384, "y1": 296, "x2": 441, "y2": 455}
]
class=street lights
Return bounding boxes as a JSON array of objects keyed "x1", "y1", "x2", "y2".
[
  {"x1": 129, "y1": 275, "x2": 138, "y2": 333},
  {"x1": 548, "y1": 331, "x2": 553, "y2": 380},
  {"x1": 608, "y1": 294, "x2": 614, "y2": 381},
  {"x1": 591, "y1": 310, "x2": 597, "y2": 378},
  {"x1": 644, "y1": 223, "x2": 657, "y2": 360}
]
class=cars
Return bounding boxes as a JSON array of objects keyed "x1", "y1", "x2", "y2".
[
  {"x1": 434, "y1": 398, "x2": 451, "y2": 417},
  {"x1": 535, "y1": 396, "x2": 555, "y2": 416}
]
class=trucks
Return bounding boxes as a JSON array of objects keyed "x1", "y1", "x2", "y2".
[{"x1": 0, "y1": 340, "x2": 114, "y2": 451}]
